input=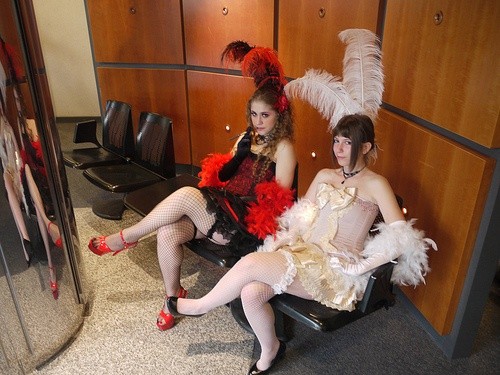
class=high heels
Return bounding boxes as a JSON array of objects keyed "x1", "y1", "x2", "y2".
[
  {"x1": 248, "y1": 340, "x2": 286, "y2": 375},
  {"x1": 23, "y1": 239, "x2": 34, "y2": 258},
  {"x1": 88, "y1": 230, "x2": 138, "y2": 256},
  {"x1": 166, "y1": 297, "x2": 205, "y2": 318},
  {"x1": 47, "y1": 222, "x2": 62, "y2": 247},
  {"x1": 156, "y1": 287, "x2": 187, "y2": 330},
  {"x1": 49, "y1": 267, "x2": 57, "y2": 292}
]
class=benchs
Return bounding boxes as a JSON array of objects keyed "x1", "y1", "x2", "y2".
[{"x1": 125, "y1": 168, "x2": 405, "y2": 351}]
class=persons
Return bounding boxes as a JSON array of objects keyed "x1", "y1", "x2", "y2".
[
  {"x1": 167, "y1": 116, "x2": 406, "y2": 375},
  {"x1": 88, "y1": 81, "x2": 298, "y2": 331},
  {"x1": 0, "y1": 34, "x2": 64, "y2": 291}
]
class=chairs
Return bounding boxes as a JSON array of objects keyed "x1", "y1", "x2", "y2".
[{"x1": 63, "y1": 98, "x2": 177, "y2": 220}]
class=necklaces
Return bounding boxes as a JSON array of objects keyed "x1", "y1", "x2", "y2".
[
  {"x1": 255, "y1": 134, "x2": 270, "y2": 146},
  {"x1": 340, "y1": 168, "x2": 363, "y2": 184}
]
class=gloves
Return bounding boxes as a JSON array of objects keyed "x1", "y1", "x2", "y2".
[
  {"x1": 198, "y1": 128, "x2": 253, "y2": 188},
  {"x1": 330, "y1": 220, "x2": 438, "y2": 287}
]
